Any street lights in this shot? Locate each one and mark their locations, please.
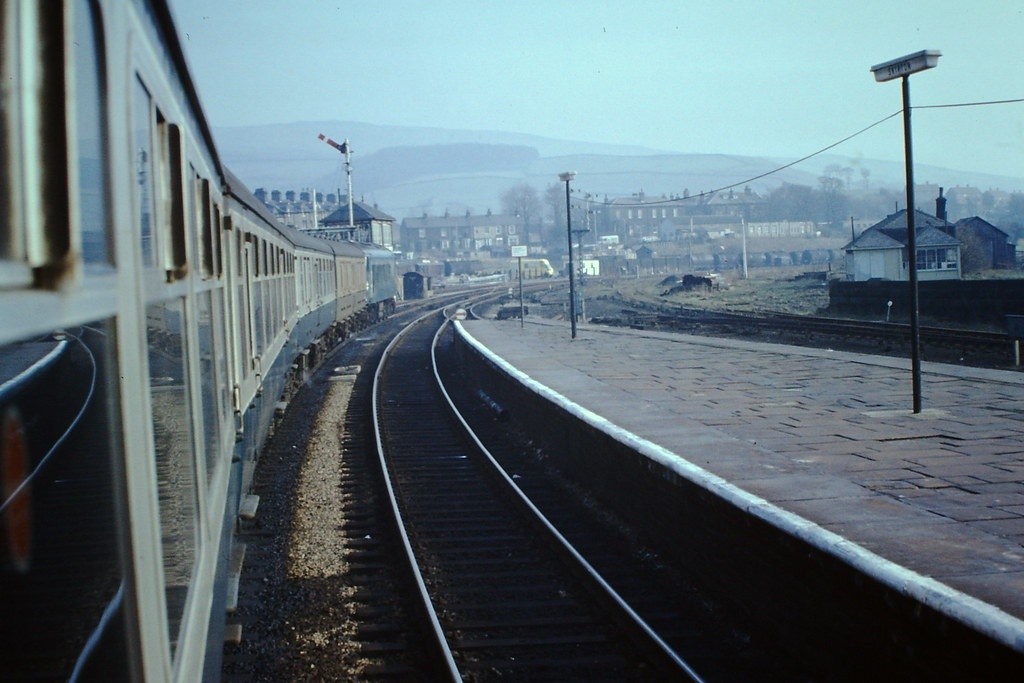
[869,49,944,414]
[557,172,578,340]
